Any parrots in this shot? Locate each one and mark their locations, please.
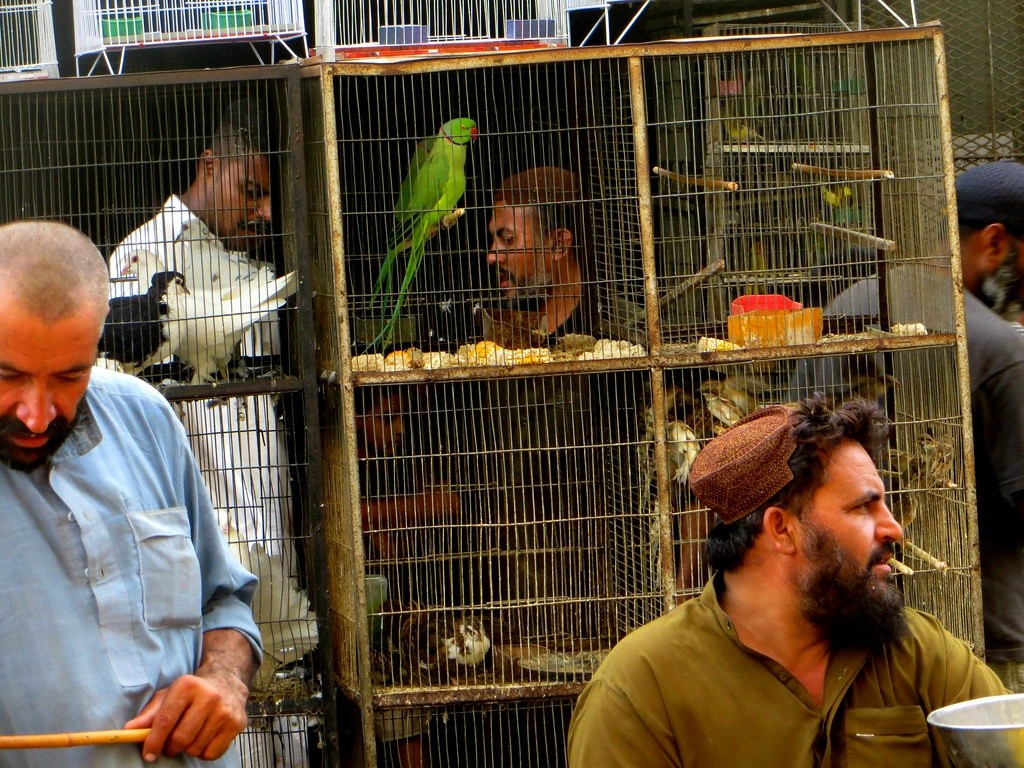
[361,117,476,356]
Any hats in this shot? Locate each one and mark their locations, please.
[495,163,585,209]
[948,158,1024,225]
[687,404,802,527]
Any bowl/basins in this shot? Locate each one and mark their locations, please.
[926,693,1024,768]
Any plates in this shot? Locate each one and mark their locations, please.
[518,649,613,676]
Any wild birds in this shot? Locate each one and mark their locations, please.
[218,514,319,696]
[370,596,527,686]
[818,185,854,209]
[632,375,777,590]
[719,108,766,144]
[827,374,955,541]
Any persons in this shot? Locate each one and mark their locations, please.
[0,220,263,768]
[566,393,1024,768]
[487,167,591,352]
[354,390,462,643]
[93,125,307,767]
[789,162,1024,696]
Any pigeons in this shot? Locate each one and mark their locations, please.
[92,218,296,382]
